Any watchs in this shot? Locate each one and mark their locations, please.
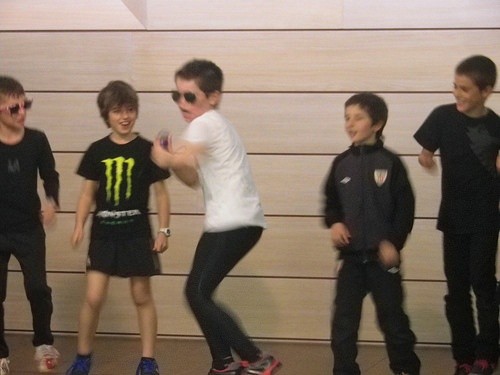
[158,228,171,237]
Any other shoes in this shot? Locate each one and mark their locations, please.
[234,352,283,375]
[136,360,159,375]
[454,360,496,375]
[208,358,239,375]
[0,358,10,375]
[67,357,91,375]
[35,345,59,372]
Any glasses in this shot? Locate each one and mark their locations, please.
[0,98,33,116]
[171,90,200,104]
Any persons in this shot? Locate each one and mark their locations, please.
[0,77,60,375]
[324,94,421,375]
[413,55,500,375]
[152,61,282,375]
[65,80,171,375]
